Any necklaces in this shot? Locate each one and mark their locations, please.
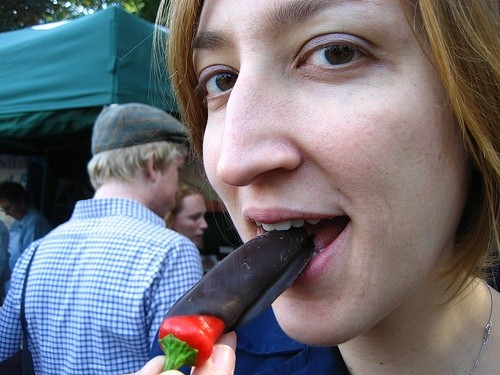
[469,276,494,375]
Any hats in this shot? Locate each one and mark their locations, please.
[91,103,189,155]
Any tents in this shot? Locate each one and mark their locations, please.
[1,6,187,156]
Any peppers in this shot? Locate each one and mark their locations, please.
[159,226,316,373]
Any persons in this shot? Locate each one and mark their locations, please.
[168,183,207,250]
[0,221,10,298]
[0,180,51,281]
[130,0,500,375]
[0,101,199,375]
